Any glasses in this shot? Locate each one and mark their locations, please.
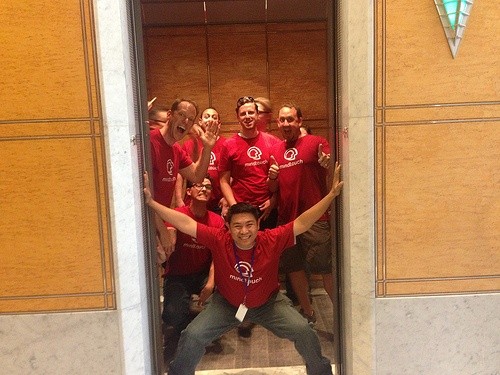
[258,111,271,115]
[189,182,212,190]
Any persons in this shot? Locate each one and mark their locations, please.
[143,160,344,375]
[143,95,344,371]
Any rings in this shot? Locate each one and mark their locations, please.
[325,164,328,167]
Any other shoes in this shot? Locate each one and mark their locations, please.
[299,308,316,323]
[206,339,224,355]
[238,328,252,339]
